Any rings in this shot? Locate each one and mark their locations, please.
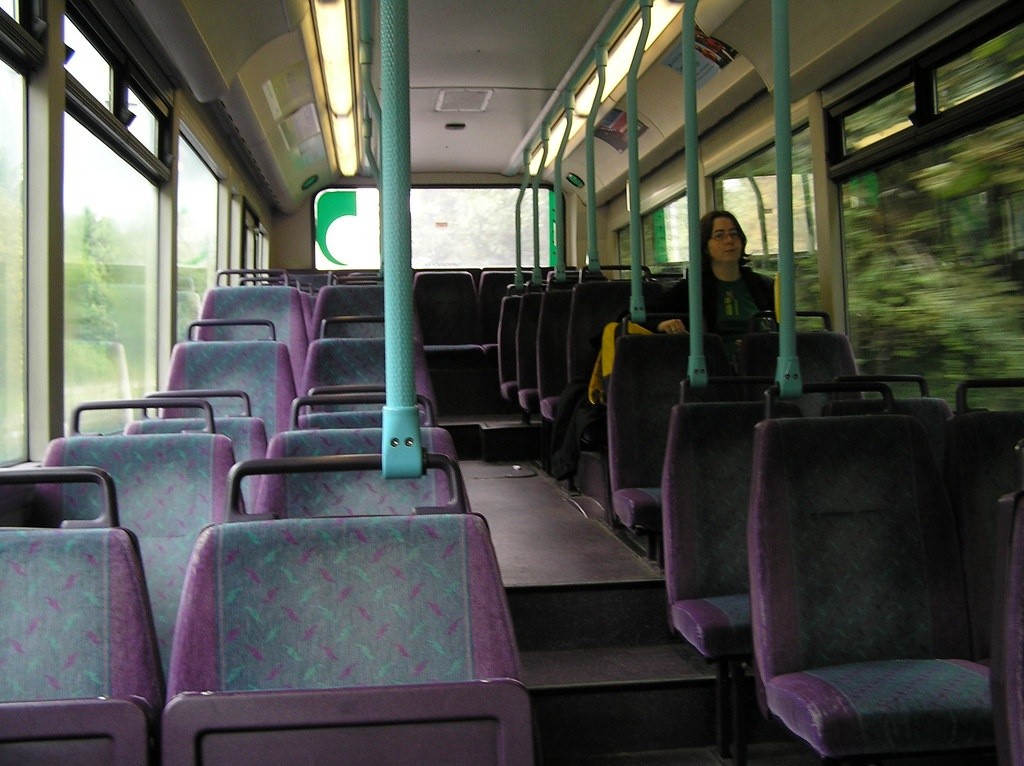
[672,328,677,333]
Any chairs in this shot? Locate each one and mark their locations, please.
[0,268,1023,766]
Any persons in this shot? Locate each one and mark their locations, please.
[649,210,777,336]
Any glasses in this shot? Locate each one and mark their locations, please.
[710,231,740,241]
